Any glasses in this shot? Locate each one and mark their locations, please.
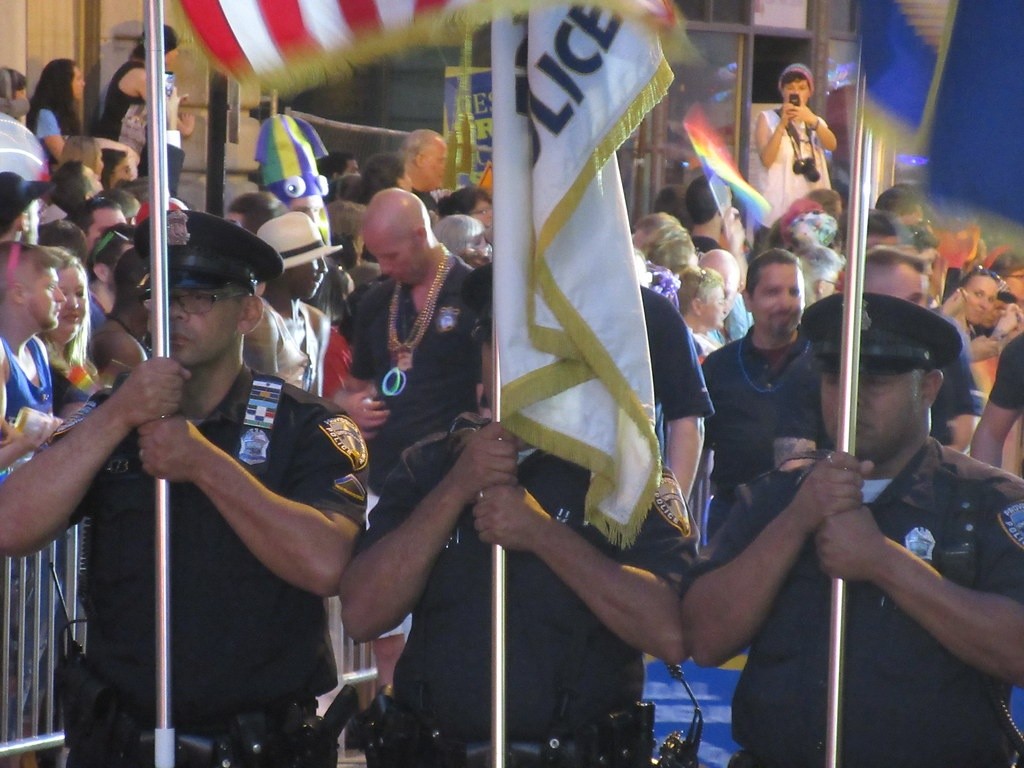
[93,229,130,264]
[970,269,998,280]
[141,294,240,315]
[468,207,493,216]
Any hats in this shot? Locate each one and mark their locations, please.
[254,115,330,204]
[462,264,493,339]
[778,63,815,96]
[0,172,55,226]
[258,211,343,268]
[801,291,963,370]
[134,209,283,296]
[141,25,179,55]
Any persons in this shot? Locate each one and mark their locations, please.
[1,26,1022,767]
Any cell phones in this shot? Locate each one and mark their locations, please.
[165,71,175,98]
[789,94,800,107]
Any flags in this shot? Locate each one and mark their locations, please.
[491,9,675,548]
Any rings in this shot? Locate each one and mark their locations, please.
[140,449,144,457]
[827,454,839,469]
[480,490,484,499]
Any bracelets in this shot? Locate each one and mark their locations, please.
[810,117,821,130]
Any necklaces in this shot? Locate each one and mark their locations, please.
[385,246,455,351]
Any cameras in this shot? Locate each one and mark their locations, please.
[793,158,821,182]
